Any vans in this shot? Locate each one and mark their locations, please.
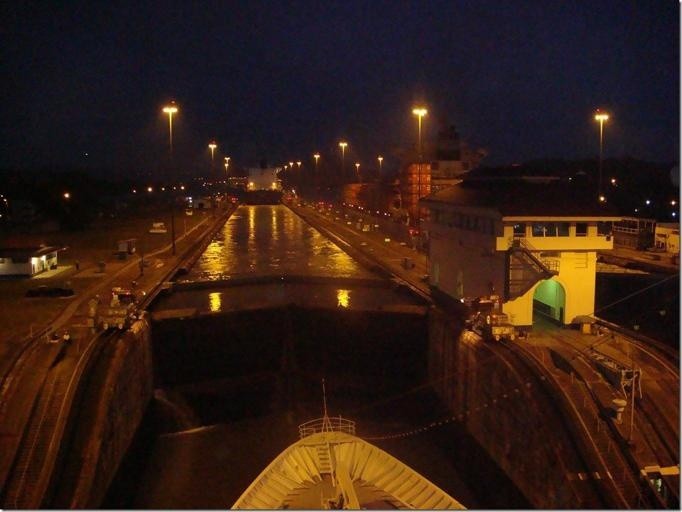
[400,256,417,269]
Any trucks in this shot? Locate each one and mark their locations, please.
[478,312,516,341]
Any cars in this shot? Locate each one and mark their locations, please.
[149,222,168,233]
[297,200,383,233]
[186,209,193,216]
[112,239,137,256]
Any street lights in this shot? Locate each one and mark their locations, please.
[355,161,360,174]
[162,107,177,255]
[224,157,230,172]
[209,138,216,180]
[413,109,427,253]
[314,151,320,166]
[378,155,383,168]
[595,113,610,189]
[276,159,301,176]
[339,143,347,171]
